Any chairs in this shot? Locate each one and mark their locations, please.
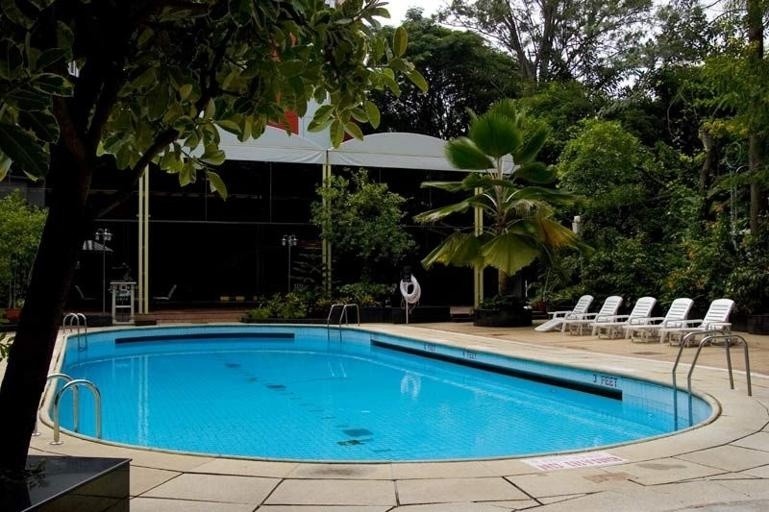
[531,294,735,344]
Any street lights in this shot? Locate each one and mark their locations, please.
[95,228,113,313]
[280,232,298,293]
